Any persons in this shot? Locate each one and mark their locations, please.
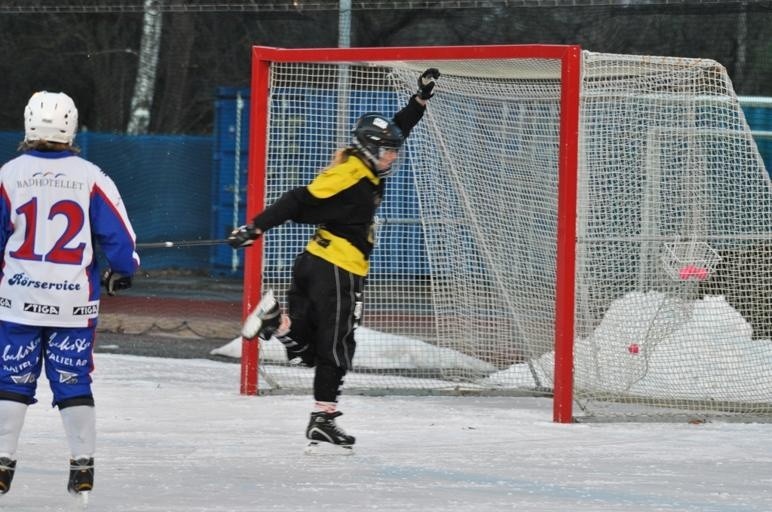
[228,67,440,443]
[0,91,142,493]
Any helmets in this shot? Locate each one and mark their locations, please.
[23,90,78,147]
[350,112,404,178]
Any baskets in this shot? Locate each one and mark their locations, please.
[663,241,721,282]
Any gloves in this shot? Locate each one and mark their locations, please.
[417,69,440,99]
[101,269,131,297]
[228,224,262,248]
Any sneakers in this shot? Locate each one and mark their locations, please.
[252,303,282,340]
[306,411,355,445]
[0,457,17,494]
[68,457,94,494]
